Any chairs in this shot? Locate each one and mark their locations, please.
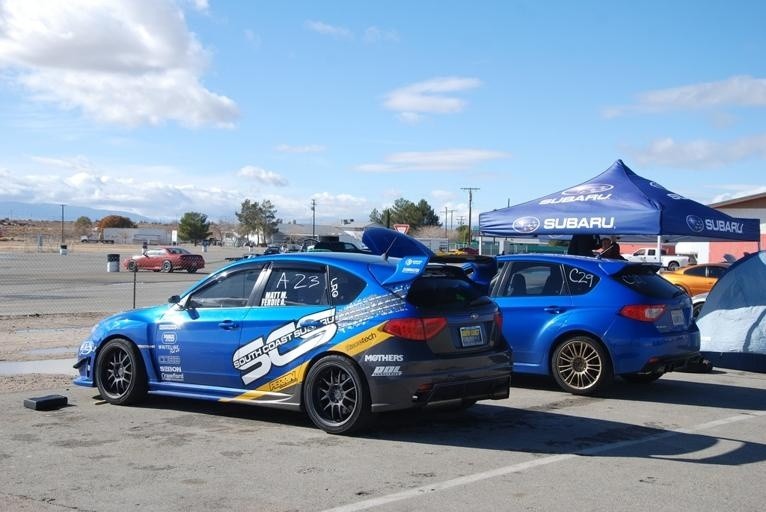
[504,272,557,297]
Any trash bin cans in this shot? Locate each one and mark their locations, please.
[202,244,208,252]
[59,245,67,256]
[106,253,121,272]
[249,246,254,252]
[141,246,147,255]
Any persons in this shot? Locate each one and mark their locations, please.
[596,238,624,260]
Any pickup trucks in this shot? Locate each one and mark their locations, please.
[627,247,697,271]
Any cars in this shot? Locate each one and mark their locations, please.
[462,252,700,395]
[658,263,734,319]
